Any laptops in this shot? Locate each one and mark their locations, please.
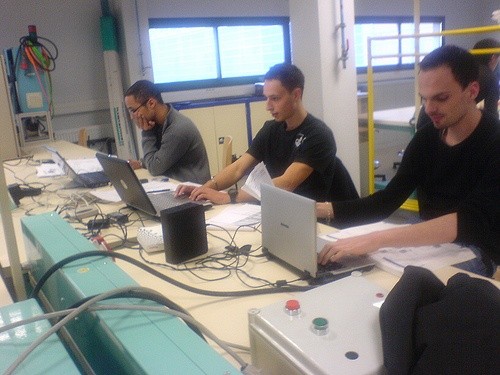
[260,183,375,277]
[41,145,111,187]
[95,152,213,218]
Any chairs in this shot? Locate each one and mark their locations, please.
[78,129,91,146]
[222,137,233,169]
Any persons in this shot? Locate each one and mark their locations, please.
[125,79,211,185]
[315,45,500,279]
[174,64,337,202]
[416,39,500,127]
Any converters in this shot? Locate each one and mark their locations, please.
[88,220,110,230]
[108,211,128,223]
[22,186,41,198]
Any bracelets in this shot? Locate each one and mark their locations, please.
[325,201,331,226]
[212,175,217,191]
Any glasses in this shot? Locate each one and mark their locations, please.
[128,98,150,116]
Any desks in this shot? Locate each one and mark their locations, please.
[0,141,500,368]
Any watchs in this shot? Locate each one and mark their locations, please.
[228,189,238,203]
